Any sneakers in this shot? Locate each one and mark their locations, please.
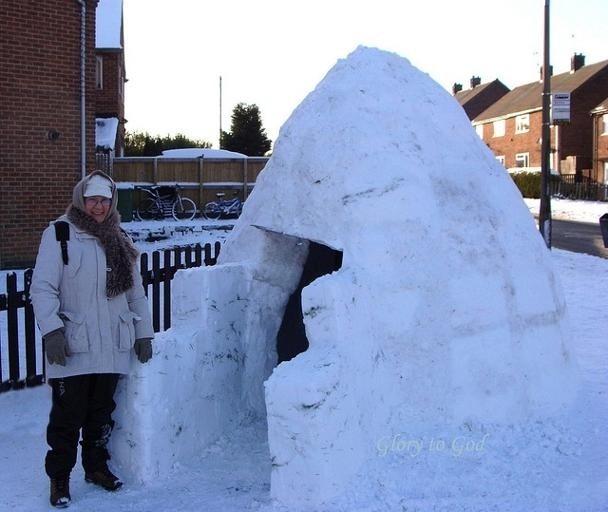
[49,477,71,508]
[84,467,122,491]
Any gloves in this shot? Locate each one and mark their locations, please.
[134,339,151,363]
[43,330,70,366]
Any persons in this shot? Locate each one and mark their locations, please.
[28,169,157,509]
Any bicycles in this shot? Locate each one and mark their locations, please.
[201,188,245,221]
[135,183,197,222]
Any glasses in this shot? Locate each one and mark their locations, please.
[85,198,111,207]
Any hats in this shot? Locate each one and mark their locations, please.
[83,174,113,199]
